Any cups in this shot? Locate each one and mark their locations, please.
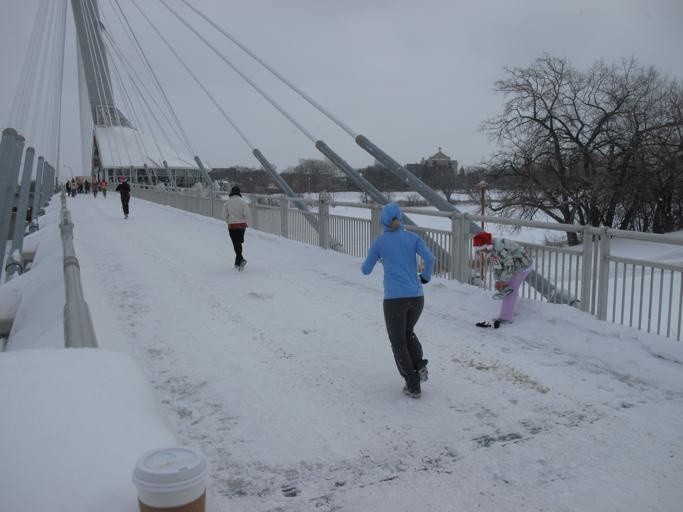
[131,444,209,511]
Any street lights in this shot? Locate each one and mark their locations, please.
[64,165,72,179]
[476,179,491,281]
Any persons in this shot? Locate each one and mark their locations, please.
[115,176,130,219]
[65,176,107,198]
[471,231,533,325]
[221,186,247,271]
[361,202,434,398]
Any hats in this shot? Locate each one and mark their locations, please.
[228,186,242,198]
[472,232,493,251]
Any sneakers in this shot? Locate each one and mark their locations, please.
[491,286,513,300]
[238,259,247,271]
[418,368,429,382]
[491,318,513,324]
[403,386,421,398]
